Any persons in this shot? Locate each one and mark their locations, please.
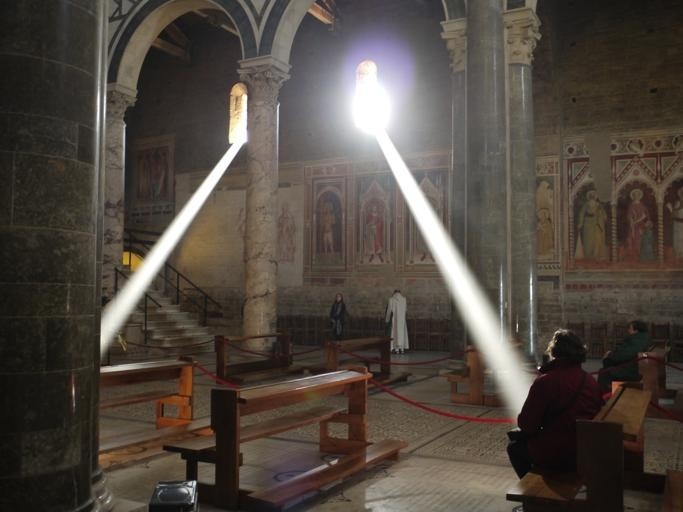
[329,293,345,340]
[385,290,410,354]
[277,202,297,262]
[320,205,336,253]
[536,180,682,260]
[506,328,606,478]
[367,205,382,252]
[598,320,651,399]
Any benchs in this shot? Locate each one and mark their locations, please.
[162,363,409,512]
[448,345,506,407]
[214,328,304,386]
[507,385,683,512]
[611,346,683,420]
[99,355,214,469]
[303,336,409,397]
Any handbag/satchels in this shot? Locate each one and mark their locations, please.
[508,428,529,441]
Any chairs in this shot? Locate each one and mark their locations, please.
[567,320,683,362]
[277,315,450,351]
